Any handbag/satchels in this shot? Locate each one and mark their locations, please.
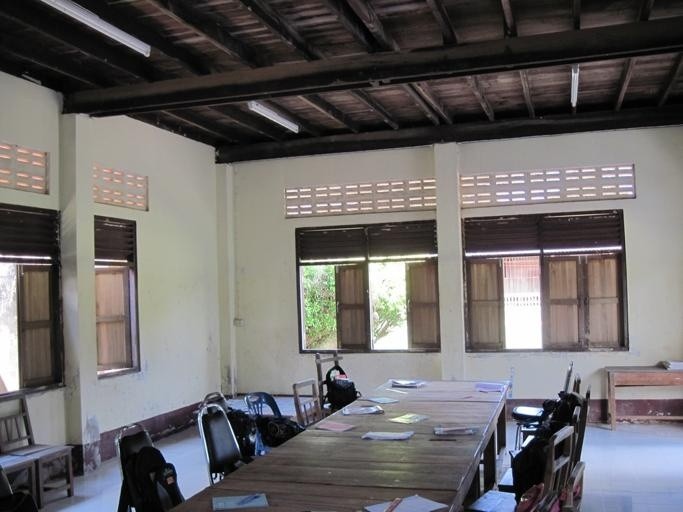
[132,445,182,512]
[508,391,583,512]
[256,413,304,447]
[327,365,357,409]
[227,409,262,458]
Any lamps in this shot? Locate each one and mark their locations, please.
[42,0,151,58]
[571,64,579,107]
[248,101,299,134]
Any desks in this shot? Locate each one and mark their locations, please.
[167,380,513,512]
[604,366,683,430]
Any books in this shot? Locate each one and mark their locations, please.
[211,491,270,511]
[360,430,415,441]
[342,405,384,415]
[432,424,478,441]
[473,381,504,392]
[662,359,682,372]
[388,413,430,424]
[314,420,353,433]
[364,495,450,512]
[390,378,427,388]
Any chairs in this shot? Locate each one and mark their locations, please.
[0,454,38,512]
[509,360,574,450]
[496,378,590,491]
[468,423,576,509]
[114,423,187,511]
[197,404,246,491]
[245,391,283,456]
[519,376,582,441]
[202,390,263,467]
[314,351,359,417]
[0,392,75,508]
[292,380,331,431]
